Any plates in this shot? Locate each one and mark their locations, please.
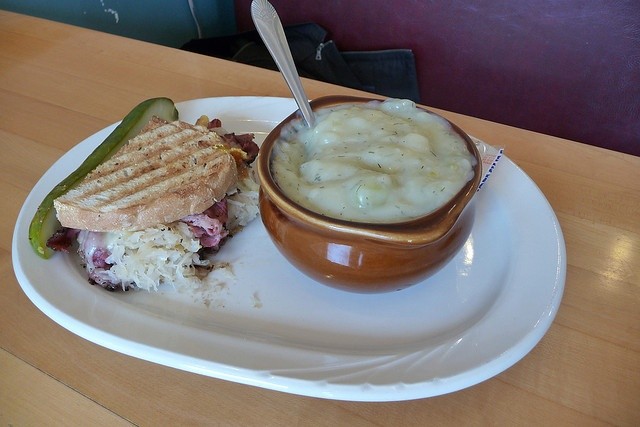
[10,94,569,404]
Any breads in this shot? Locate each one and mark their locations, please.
[53,119,235,231]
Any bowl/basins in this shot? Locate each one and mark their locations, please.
[256,97,481,296]
